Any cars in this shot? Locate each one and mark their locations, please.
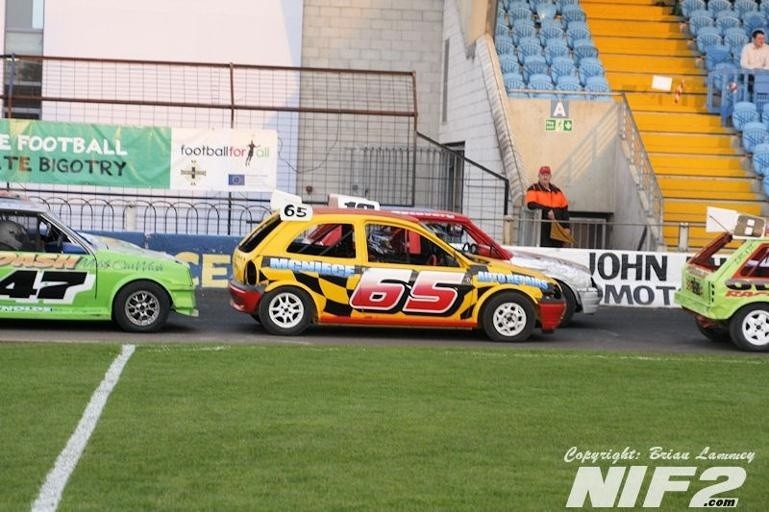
[228,189,564,343]
[677,234,769,351]
[0,190,201,334]
[325,194,603,325]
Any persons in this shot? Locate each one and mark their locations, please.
[1,219,46,254]
[367,230,399,263]
[526,165,572,247]
[737,30,768,96]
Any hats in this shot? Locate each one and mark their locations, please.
[539,166,551,175]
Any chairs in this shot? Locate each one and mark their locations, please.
[495,0,614,101]
[681,0,769,201]
